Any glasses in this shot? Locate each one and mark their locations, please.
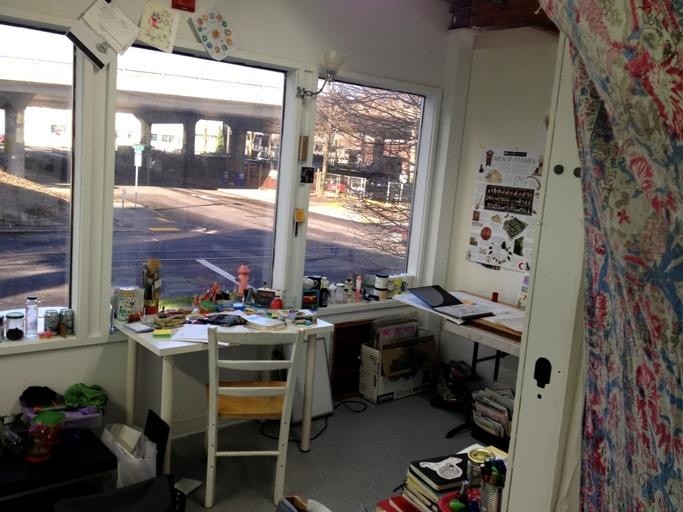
[295,50,346,102]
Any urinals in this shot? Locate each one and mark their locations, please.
[153,329,172,338]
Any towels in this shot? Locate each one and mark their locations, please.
[221,171,245,186]
[104,423,153,486]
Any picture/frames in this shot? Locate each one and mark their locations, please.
[113,308,336,476]
[442,295,523,439]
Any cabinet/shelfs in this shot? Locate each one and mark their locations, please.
[335,278,357,304]
[25,295,39,340]
[466,449,496,487]
[6,311,24,341]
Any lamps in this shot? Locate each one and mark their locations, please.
[203,322,307,508]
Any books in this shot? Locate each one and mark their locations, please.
[354,334,435,406]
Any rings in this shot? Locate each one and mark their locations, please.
[480,458,505,493]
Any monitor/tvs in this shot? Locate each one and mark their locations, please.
[472,383,514,438]
[171,314,287,346]
[111,424,148,458]
[408,285,496,320]
[376,443,469,512]
